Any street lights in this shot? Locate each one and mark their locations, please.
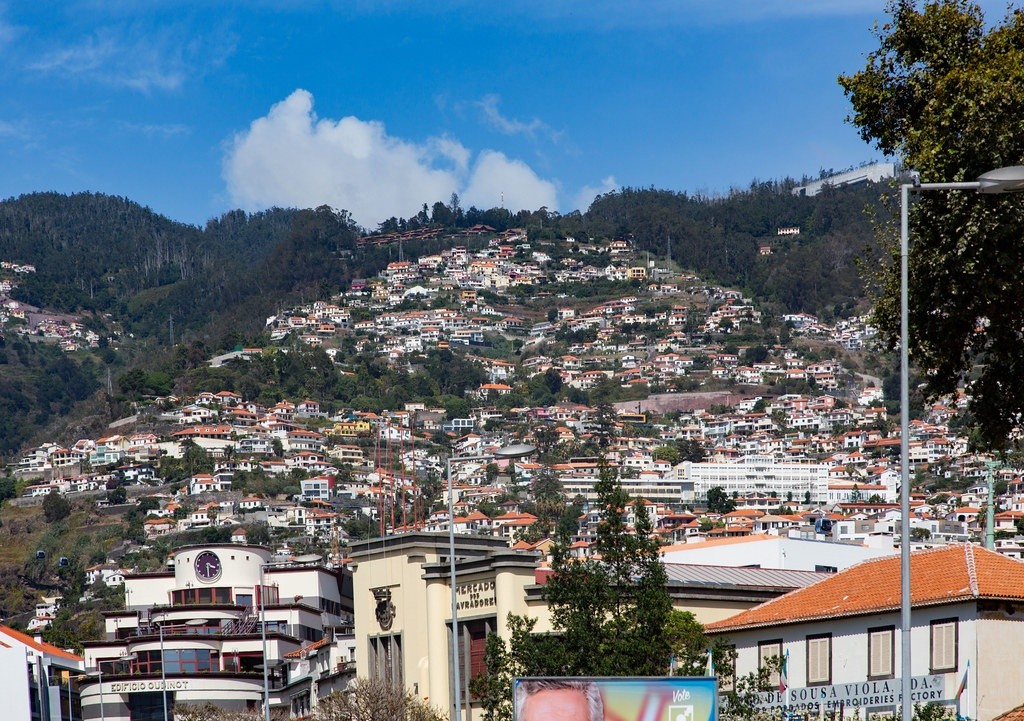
[901,164,1024,721]
[99,656,137,721]
[160,619,209,721]
[259,555,324,721]
[68,671,104,721]
[448,445,538,721]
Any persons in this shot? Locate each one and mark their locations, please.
[517,679,603,721]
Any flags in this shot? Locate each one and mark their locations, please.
[954,670,967,700]
[779,658,787,694]
